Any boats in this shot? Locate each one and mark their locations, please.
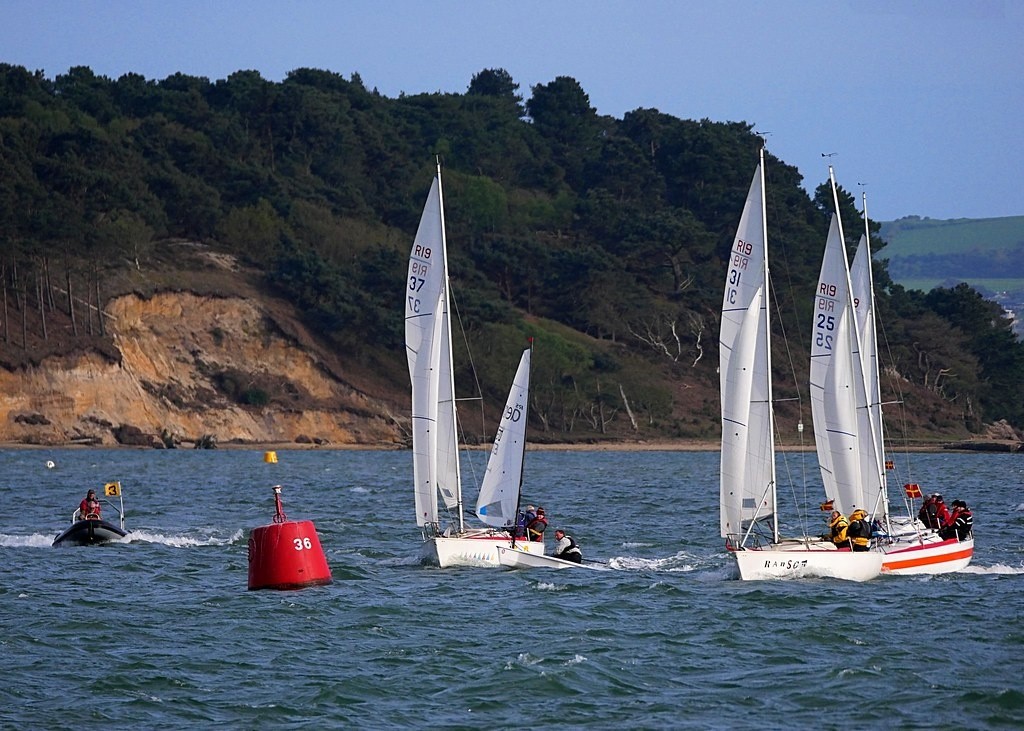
[50,499,135,549]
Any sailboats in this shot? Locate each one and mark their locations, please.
[474,334,615,574]
[405,152,548,567]
[717,140,887,582]
[808,157,976,579]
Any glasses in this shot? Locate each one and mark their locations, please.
[936,499,942,502]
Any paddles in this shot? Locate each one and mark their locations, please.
[544,553,607,564]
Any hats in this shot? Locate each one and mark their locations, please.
[958,501,966,509]
[951,500,960,506]
[537,507,544,515]
[932,492,940,498]
[526,505,535,511]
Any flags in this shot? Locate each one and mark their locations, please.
[904,484,923,498]
[105,482,121,496]
[820,500,833,511]
[885,461,895,470]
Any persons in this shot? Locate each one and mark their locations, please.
[518,509,527,528]
[79,489,101,519]
[939,499,973,541]
[919,492,950,528]
[525,507,547,541]
[525,505,537,526]
[823,511,850,548]
[552,529,582,564]
[846,510,872,552]
